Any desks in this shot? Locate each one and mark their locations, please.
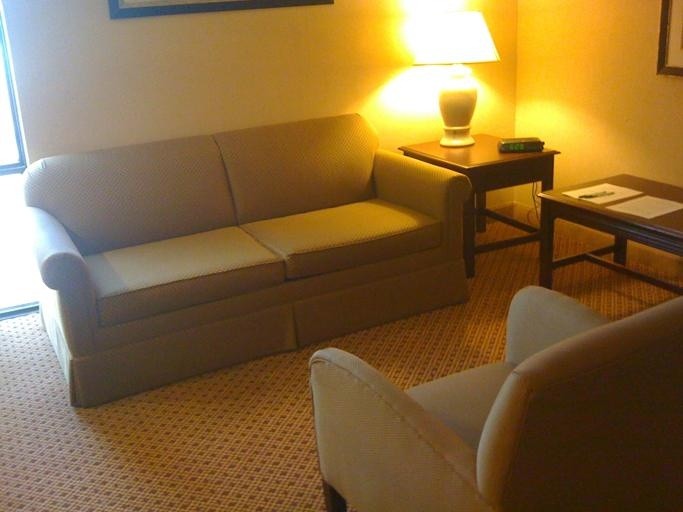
[399,132,560,279]
[537,175,683,296]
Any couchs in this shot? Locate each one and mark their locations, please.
[309,286,682,512]
[22,113,472,409]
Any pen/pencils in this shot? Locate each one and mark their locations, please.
[589,192,615,197]
[579,190,607,198]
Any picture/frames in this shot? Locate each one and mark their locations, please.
[107,0,333,19]
[655,0,683,76]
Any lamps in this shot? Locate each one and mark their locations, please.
[413,12,501,148]
